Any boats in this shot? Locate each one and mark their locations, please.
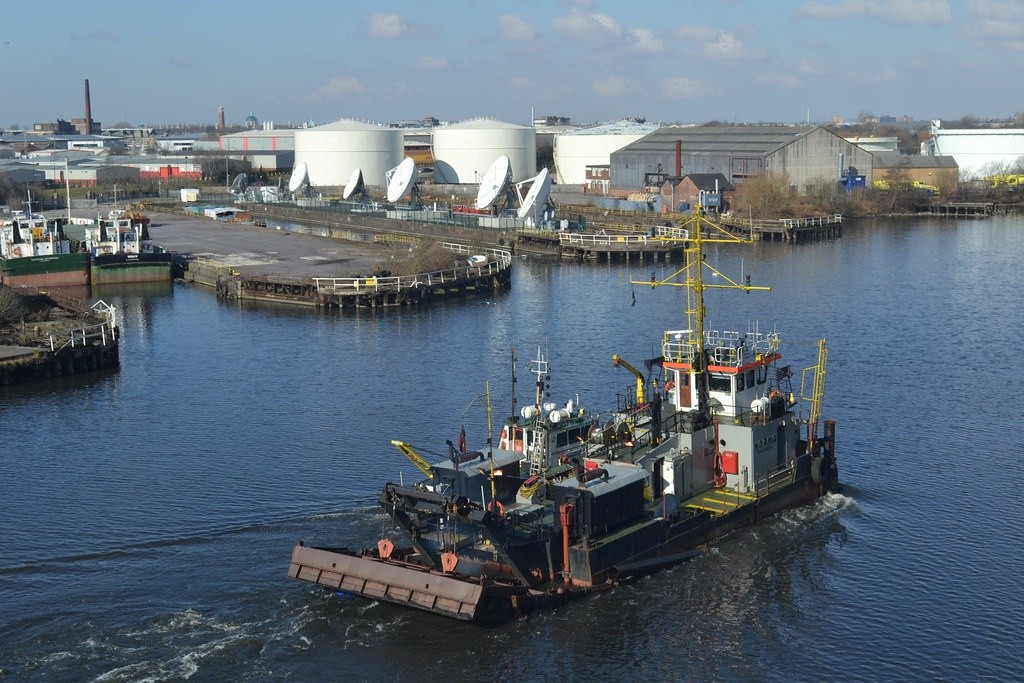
[0,189,90,290]
[48,155,96,226]
[285,189,843,625]
[83,182,175,284]
